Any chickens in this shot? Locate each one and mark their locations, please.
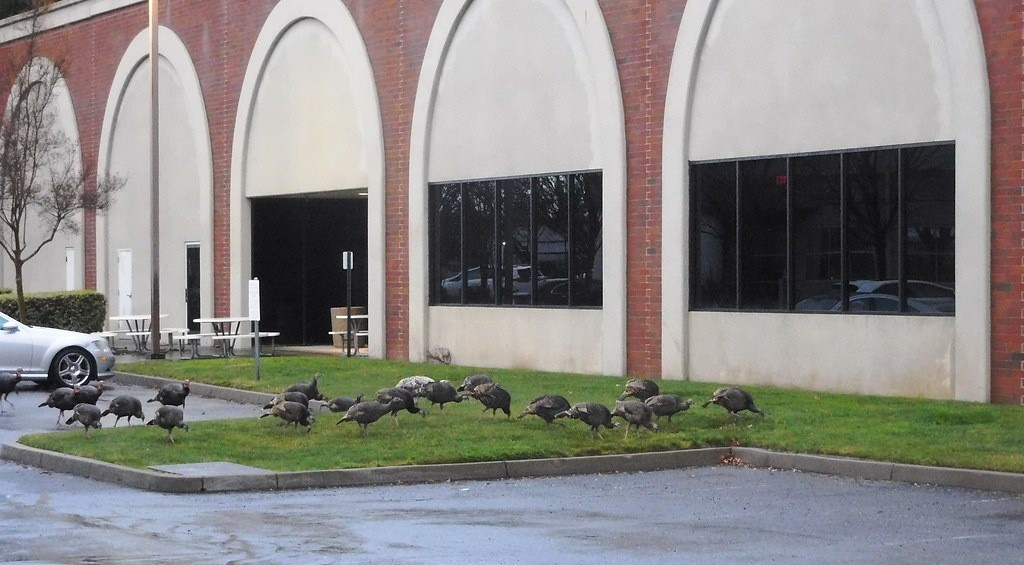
[38,379,191,444]
[702,386,765,418]
[0,367,24,411]
[257,371,697,440]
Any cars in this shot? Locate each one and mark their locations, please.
[0,312,116,390]
[502,279,603,306]
[793,293,944,315]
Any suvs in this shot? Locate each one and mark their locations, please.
[440,266,545,299]
[833,278,955,308]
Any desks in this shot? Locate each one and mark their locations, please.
[336,315,368,356]
[109,314,170,353]
[193,317,249,357]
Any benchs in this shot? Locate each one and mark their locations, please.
[92,328,280,339]
[328,330,369,336]
